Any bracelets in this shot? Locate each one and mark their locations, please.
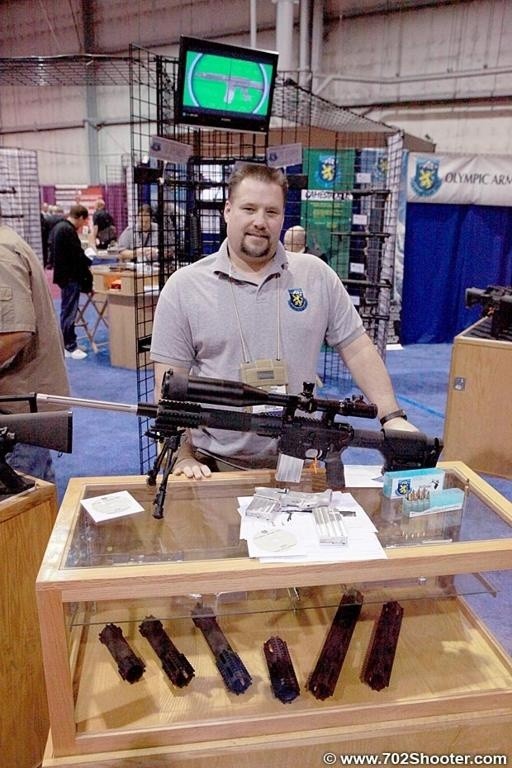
[376,405,412,425]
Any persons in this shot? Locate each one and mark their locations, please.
[40,203,160,264]
[50,204,99,355]
[0,221,72,483]
[151,161,423,480]
[282,225,329,265]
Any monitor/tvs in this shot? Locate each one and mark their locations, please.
[173,35,279,136]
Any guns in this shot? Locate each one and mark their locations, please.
[30,370,442,516]
[0,394,73,458]
[185,68,270,104]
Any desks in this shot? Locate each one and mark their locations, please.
[442,314,511,480]
[108,290,158,371]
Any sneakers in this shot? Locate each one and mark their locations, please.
[77,345,87,351]
[64,348,87,359]
